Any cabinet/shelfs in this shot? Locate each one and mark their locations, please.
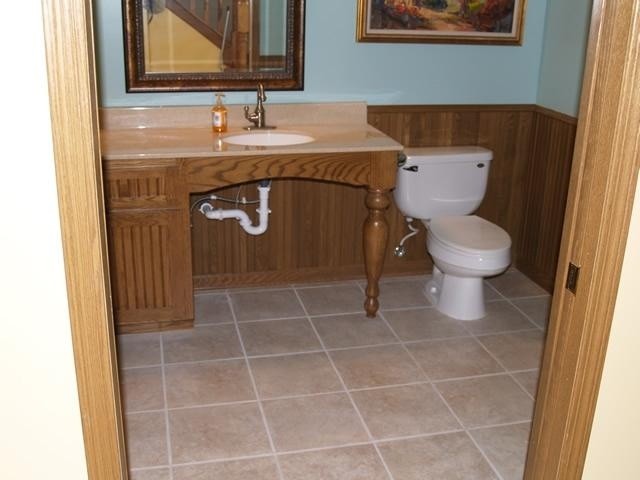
[103,158,194,335]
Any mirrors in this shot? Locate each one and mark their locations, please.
[120,1,306,93]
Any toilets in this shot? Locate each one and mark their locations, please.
[393,146,513,321]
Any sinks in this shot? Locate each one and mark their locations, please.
[218,129,317,147]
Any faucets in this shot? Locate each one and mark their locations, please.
[245,83,267,128]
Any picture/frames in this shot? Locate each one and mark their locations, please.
[356,0,526,46]
